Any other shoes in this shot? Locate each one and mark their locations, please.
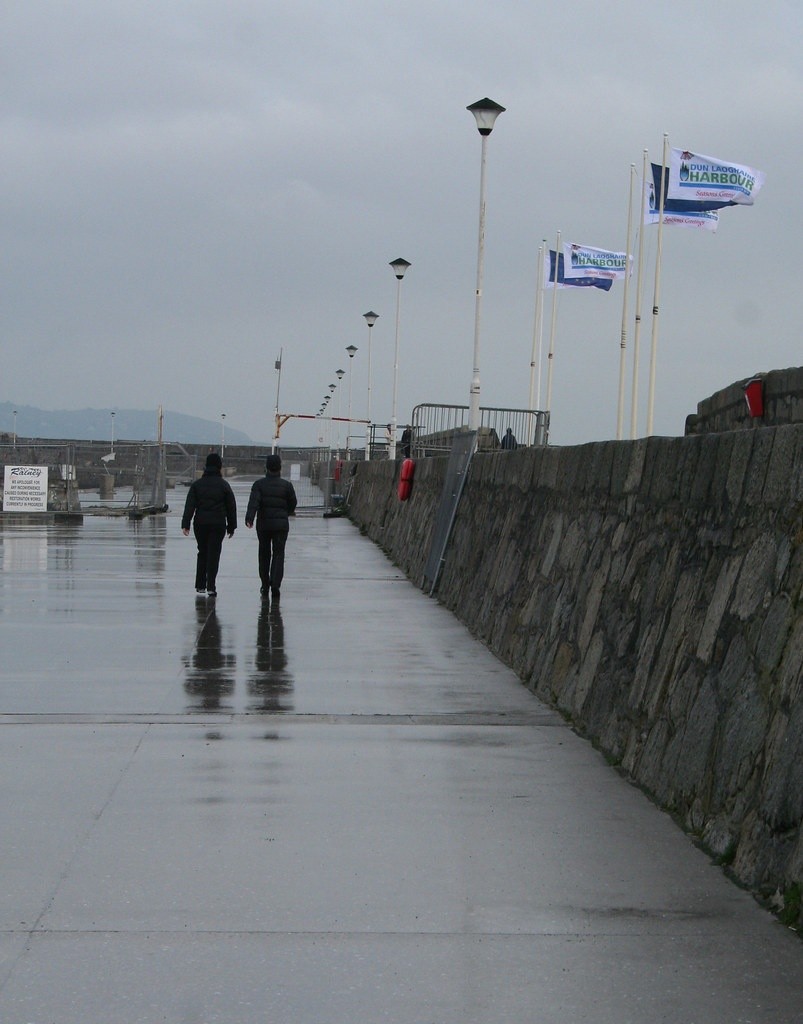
[196,588,206,593]
[260,587,269,596]
[207,587,217,595]
[271,583,280,597]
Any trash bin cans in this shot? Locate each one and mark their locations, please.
[331,494,344,512]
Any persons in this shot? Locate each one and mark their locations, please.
[402,424,413,458]
[489,429,499,449]
[501,428,518,449]
[385,424,398,454]
[245,455,297,597]
[181,454,236,596]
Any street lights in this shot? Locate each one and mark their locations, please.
[220,413,226,457]
[363,309,381,461]
[110,411,115,445]
[314,342,359,461]
[12,410,18,446]
[387,257,413,459]
[465,96,508,431]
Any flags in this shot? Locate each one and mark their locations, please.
[562,241,634,280]
[649,162,669,212]
[544,249,613,292]
[642,181,720,232]
[663,146,765,211]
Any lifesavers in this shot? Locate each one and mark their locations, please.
[298,449,304,455]
[335,459,343,481]
[319,437,324,442]
[398,459,413,500]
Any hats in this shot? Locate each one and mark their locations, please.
[205,453,222,469]
[266,455,281,472]
